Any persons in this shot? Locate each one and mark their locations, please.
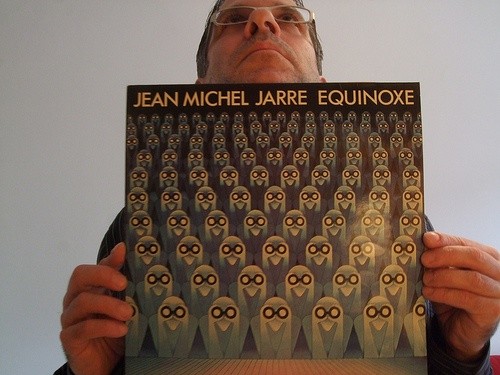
[51,0,500,375]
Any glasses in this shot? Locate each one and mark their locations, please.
[205,4,317,58]
